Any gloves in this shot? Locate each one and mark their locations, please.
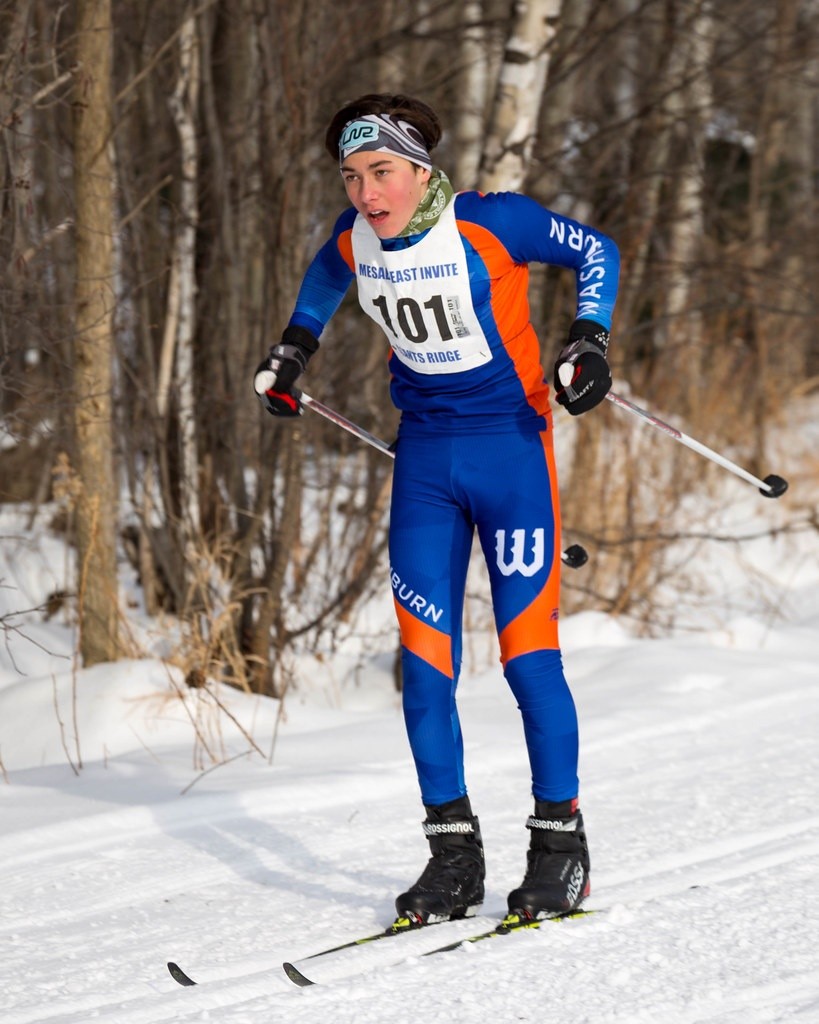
[554,319,613,416]
[253,326,319,417]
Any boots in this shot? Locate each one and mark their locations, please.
[395,795,486,924]
[507,796,590,918]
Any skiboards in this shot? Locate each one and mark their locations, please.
[167,908,605,988]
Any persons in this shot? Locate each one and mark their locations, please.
[255,95,620,917]
